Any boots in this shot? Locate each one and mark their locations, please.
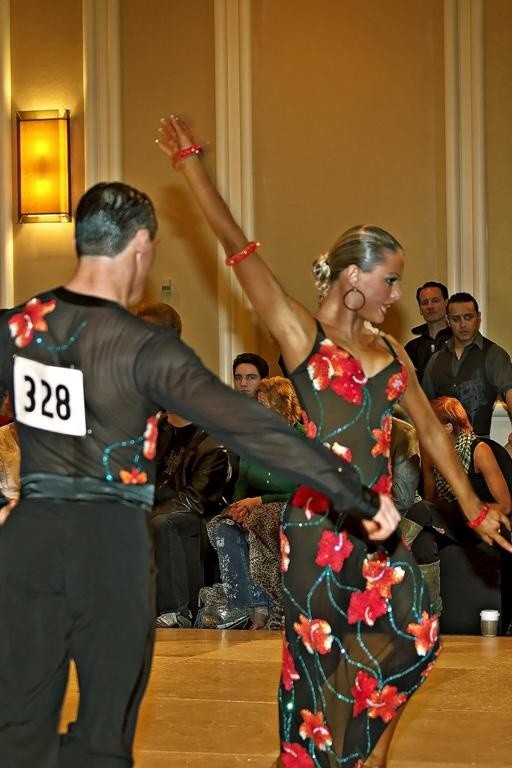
[398,516,424,548]
[417,558,443,616]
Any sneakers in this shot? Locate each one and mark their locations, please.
[155,608,192,628]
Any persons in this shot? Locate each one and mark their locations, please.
[1,182,404,768]
[154,113,512,767]
[1,281,511,639]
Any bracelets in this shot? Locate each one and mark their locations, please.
[170,140,208,171]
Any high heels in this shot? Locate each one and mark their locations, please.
[216,614,252,629]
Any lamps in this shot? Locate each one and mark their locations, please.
[16,108,73,224]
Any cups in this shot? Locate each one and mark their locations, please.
[480,610,500,637]
